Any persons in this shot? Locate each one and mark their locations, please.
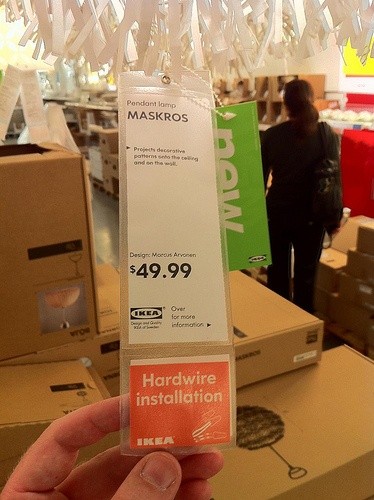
[257,80,341,314]
[0,395,224,500]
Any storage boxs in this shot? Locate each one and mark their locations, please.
[0,143,100,362]
[241,215,374,345]
[225,270,325,397]
[0,262,121,399]
[206,345,374,500]
[89,128,119,194]
[0,357,113,490]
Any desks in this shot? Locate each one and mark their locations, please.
[6,89,114,135]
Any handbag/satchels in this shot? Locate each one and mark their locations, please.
[309,121,342,232]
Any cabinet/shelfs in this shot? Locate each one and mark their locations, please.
[212,76,327,133]
